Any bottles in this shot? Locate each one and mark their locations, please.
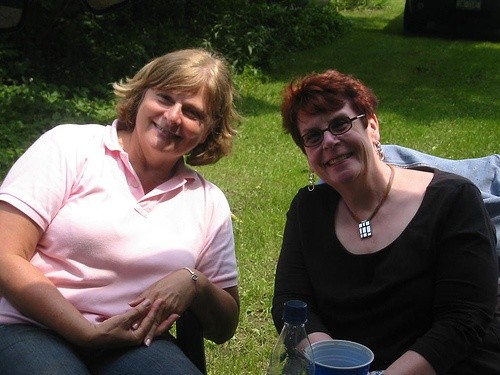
[267,300,315,375]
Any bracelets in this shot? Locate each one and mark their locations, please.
[185,267,200,292]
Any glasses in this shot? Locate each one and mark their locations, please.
[299,113,367,147]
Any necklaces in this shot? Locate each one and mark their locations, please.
[344,163,396,240]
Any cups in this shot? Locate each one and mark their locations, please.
[306,340,374,375]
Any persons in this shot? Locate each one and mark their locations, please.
[272,71,500,375]
[0,47,244,375]
